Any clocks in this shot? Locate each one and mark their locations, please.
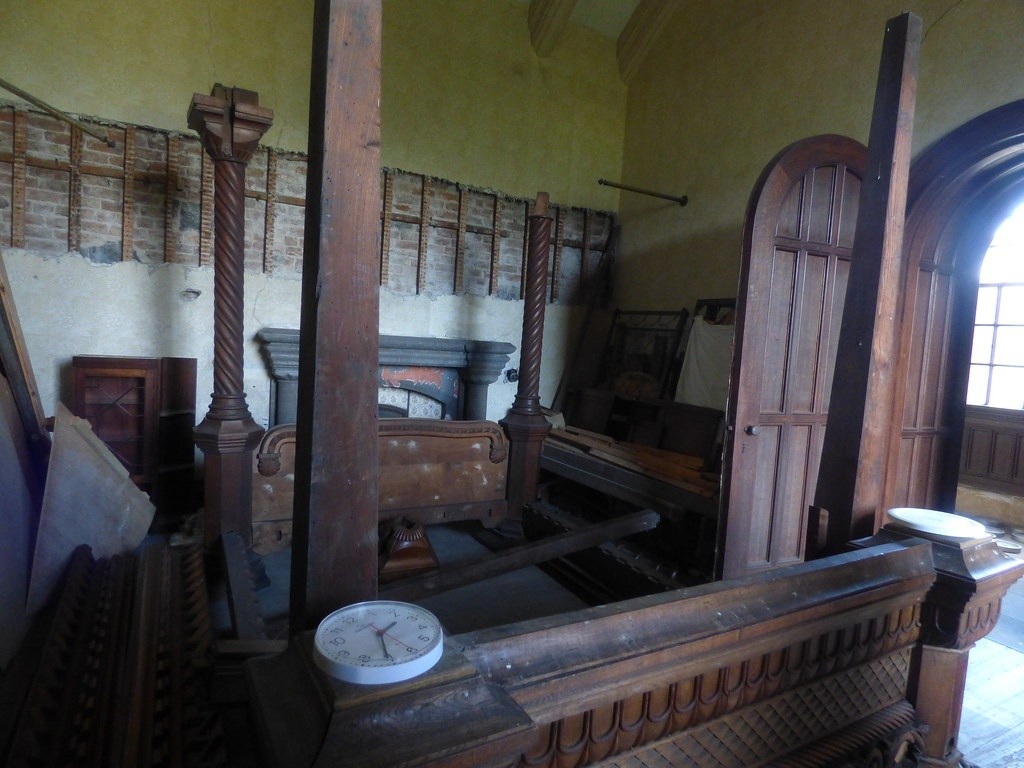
[314,599,444,686]
